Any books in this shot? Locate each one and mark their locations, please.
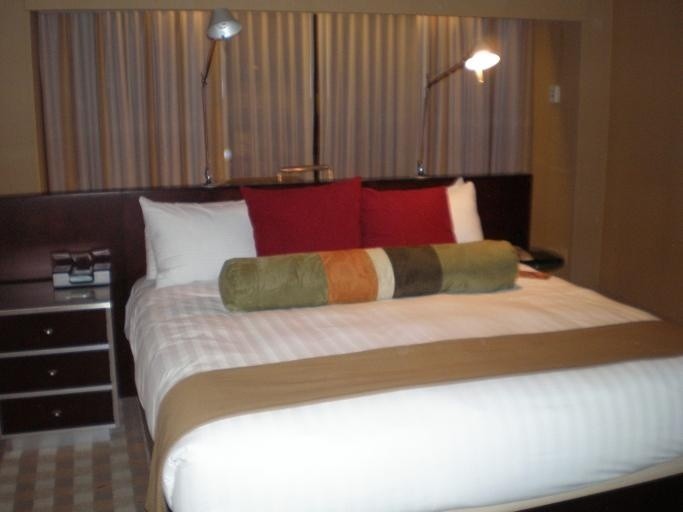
[513,245,536,262]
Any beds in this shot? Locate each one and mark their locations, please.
[1,174,683,512]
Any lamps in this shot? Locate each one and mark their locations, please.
[415,39,501,176]
[198,7,243,189]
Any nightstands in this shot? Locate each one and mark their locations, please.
[2,279,122,450]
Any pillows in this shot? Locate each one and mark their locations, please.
[138,177,519,312]
